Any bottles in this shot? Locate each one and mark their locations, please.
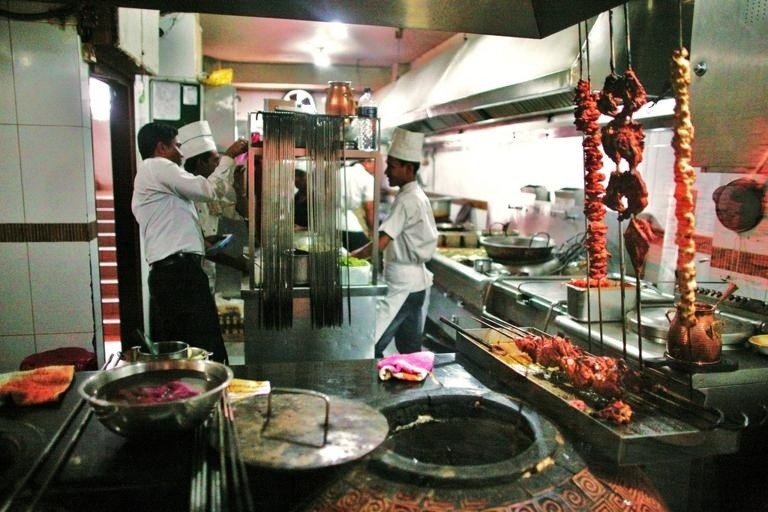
[357,87,377,151]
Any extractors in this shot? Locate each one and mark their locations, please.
[371,0,687,142]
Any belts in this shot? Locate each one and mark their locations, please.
[166,253,202,261]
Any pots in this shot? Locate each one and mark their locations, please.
[713,176,768,234]
[189,347,213,361]
[284,248,310,288]
[430,197,452,222]
[479,222,557,262]
[137,340,190,360]
[437,222,465,231]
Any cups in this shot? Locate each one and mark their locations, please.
[474,259,491,273]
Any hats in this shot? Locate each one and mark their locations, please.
[387,128,425,162]
[178,120,216,160]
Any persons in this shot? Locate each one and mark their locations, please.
[347,126,438,360]
[236,148,384,273]
[131,121,249,363]
[177,119,248,295]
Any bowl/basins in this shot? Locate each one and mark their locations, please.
[77,357,234,439]
[748,334,768,355]
